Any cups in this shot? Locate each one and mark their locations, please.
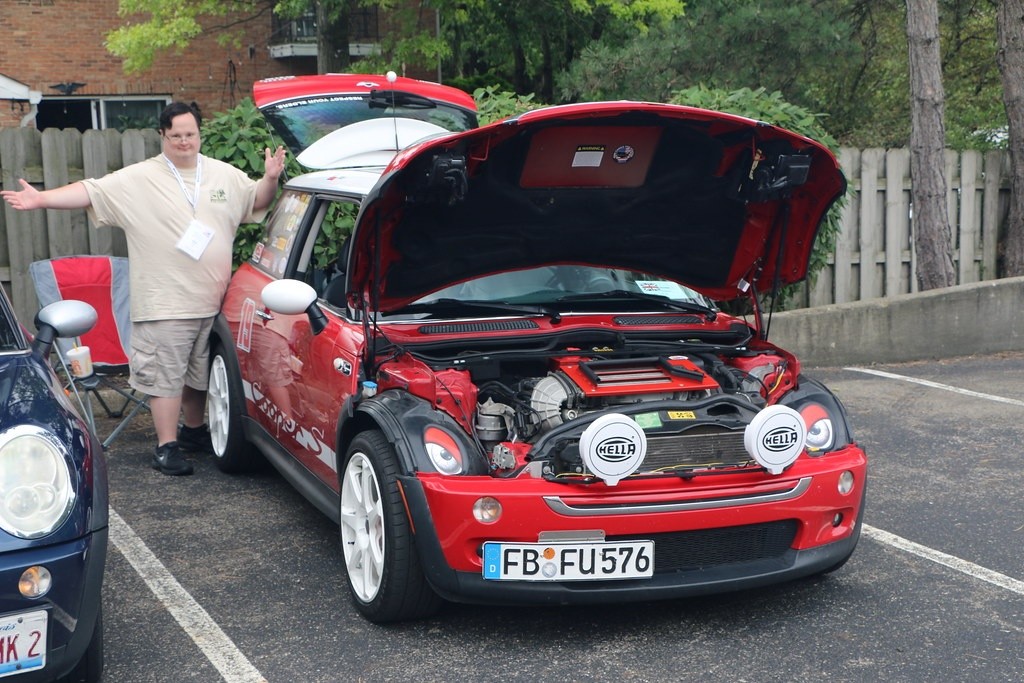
[66,346,94,379]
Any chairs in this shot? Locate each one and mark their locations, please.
[30,255,183,452]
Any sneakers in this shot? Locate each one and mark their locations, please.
[152,441,194,475]
[177,423,214,452]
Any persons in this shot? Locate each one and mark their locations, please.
[0,101,286,475]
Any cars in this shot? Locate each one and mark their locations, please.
[205,70,869,622]
[0,282,109,682]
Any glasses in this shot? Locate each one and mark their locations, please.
[162,132,201,143]
